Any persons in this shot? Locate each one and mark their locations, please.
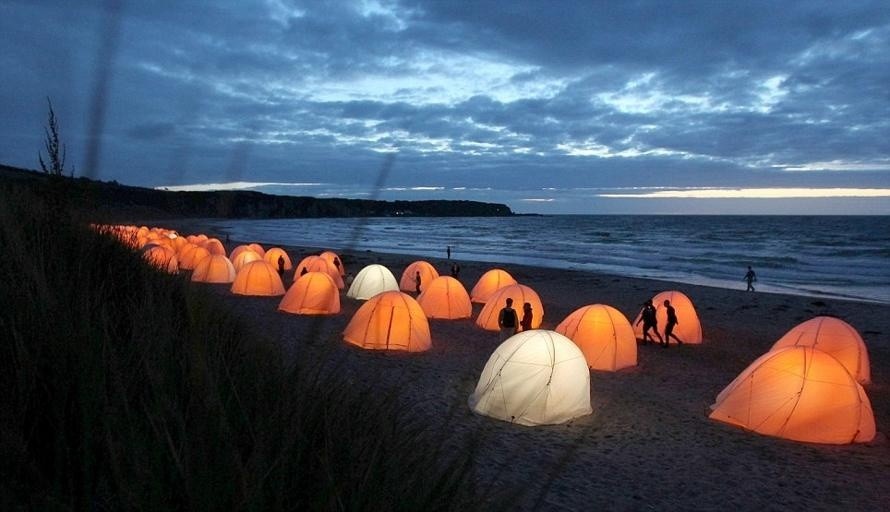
[520,302,533,333]
[645,299,663,344]
[636,303,656,344]
[446,245,451,258]
[741,266,756,292]
[333,257,340,269]
[450,259,460,277]
[498,298,519,344]
[416,271,422,294]
[664,300,684,345]
[301,267,307,276]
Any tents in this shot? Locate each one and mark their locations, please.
[319,251,346,276]
[708,344,875,448]
[346,264,399,301]
[769,315,873,387]
[277,272,342,317]
[340,291,433,354]
[474,284,545,332]
[88,222,294,297]
[633,290,704,347]
[415,276,473,320]
[293,255,345,292]
[470,269,519,304]
[553,304,638,371]
[467,329,594,427]
[400,260,440,294]
